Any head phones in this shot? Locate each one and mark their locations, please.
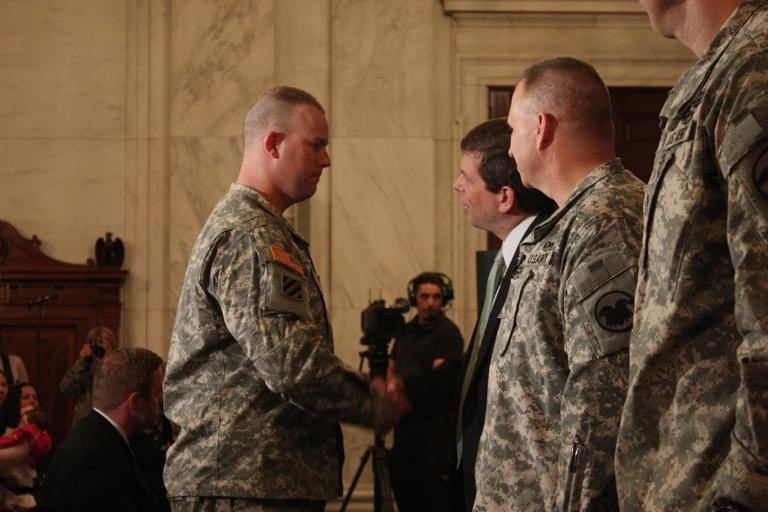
[408,273,454,307]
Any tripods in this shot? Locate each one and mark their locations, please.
[341,342,405,512]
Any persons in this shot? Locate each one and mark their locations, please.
[456,122,558,511]
[160,88,400,512]
[385,272,469,512]
[470,56,650,511]
[614,1,768,512]
[0,326,174,511]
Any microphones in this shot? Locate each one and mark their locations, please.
[430,302,452,317]
[28,292,59,304]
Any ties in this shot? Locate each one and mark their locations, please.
[455,250,504,472]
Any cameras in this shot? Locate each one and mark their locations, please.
[86,345,105,363]
[28,409,49,429]
